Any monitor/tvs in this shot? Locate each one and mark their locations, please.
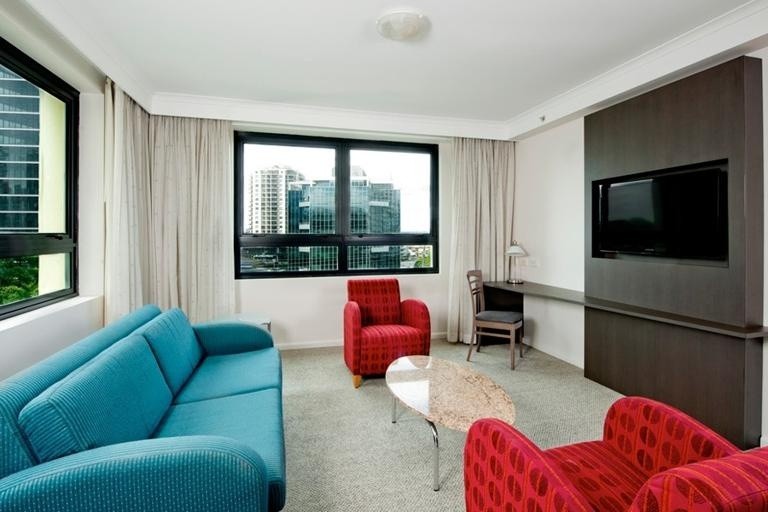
[597,168,727,260]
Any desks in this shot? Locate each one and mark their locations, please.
[477,279,768,451]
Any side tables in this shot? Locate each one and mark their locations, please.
[213,313,271,333]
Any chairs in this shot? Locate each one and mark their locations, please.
[466,270,524,370]
[344,278,430,388]
[463,395,768,512]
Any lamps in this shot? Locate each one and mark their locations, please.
[504,241,525,284]
[377,11,431,42]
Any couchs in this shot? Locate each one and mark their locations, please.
[0,305,285,512]
